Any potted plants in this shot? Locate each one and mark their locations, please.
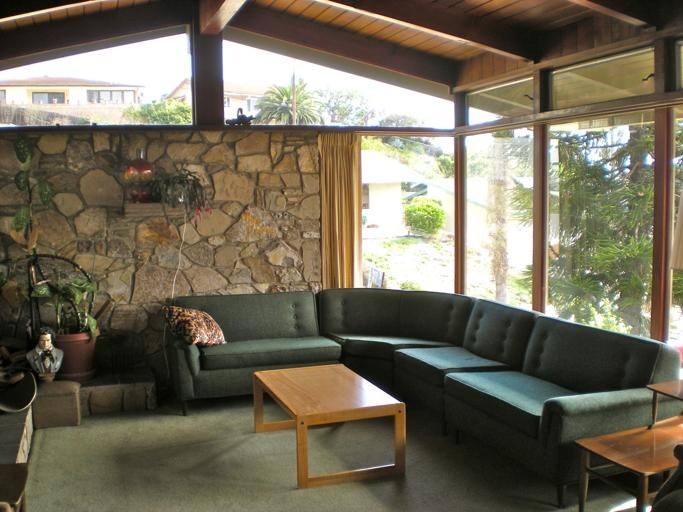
[150,167,210,227]
[34,271,111,374]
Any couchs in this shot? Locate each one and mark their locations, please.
[159,288,347,415]
[390,295,683,511]
[313,282,479,419]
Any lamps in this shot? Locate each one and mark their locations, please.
[123,154,154,204]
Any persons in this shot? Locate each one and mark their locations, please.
[25,325,64,374]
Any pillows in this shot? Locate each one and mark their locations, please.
[161,298,228,350]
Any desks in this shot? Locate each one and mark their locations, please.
[250,364,411,492]
[571,370,683,511]
